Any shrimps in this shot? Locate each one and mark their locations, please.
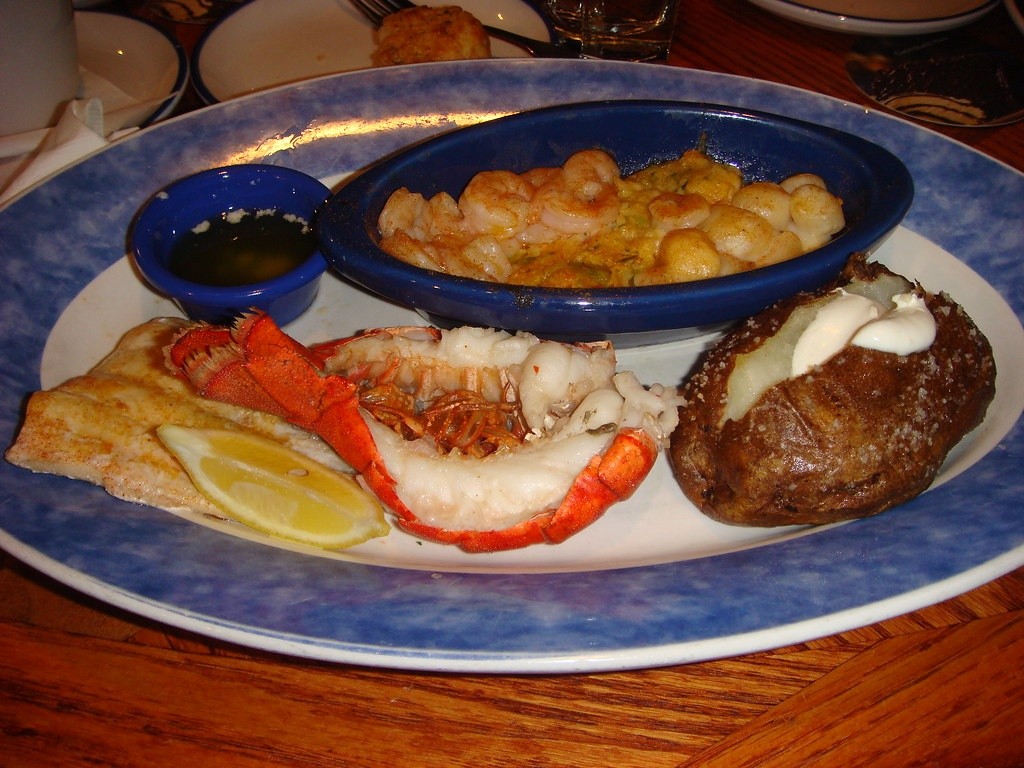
[375,148,847,288]
[161,303,684,554]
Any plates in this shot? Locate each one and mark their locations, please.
[321,101,913,351]
[1004,0,1024,29]
[192,0,559,107]
[0,58,1024,677]
[844,38,1023,127]
[150,0,226,24]
[0,7,190,128]
[754,0,1001,35]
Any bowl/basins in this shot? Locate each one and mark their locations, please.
[128,164,332,327]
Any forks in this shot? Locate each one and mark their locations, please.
[348,0,559,59]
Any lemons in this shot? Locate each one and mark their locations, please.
[157,424,393,549]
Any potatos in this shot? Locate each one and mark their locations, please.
[667,262,998,526]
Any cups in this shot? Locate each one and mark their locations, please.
[0,0,82,138]
[541,0,680,65]
